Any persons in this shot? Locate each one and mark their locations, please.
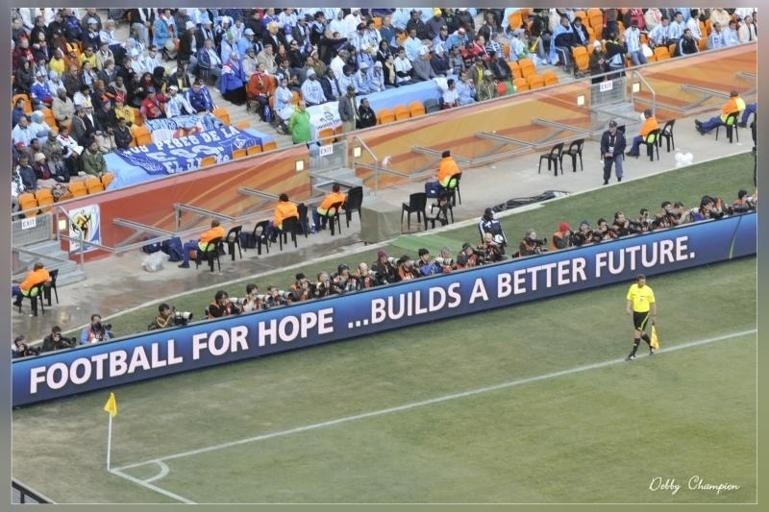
[11,6,758,358]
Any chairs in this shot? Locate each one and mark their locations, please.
[42,268,60,304]
[18,281,46,316]
